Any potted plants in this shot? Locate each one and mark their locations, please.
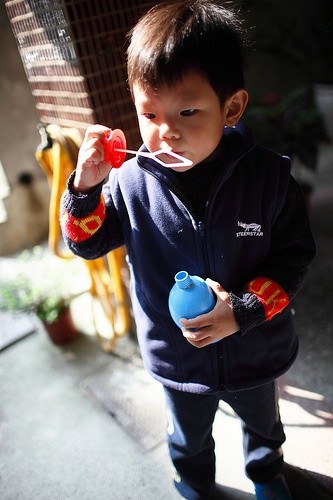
[1,274,79,346]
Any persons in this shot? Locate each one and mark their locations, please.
[59,0,318,500]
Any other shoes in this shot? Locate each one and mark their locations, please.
[254,473,292,500]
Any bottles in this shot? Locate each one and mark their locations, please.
[168,272,221,343]
